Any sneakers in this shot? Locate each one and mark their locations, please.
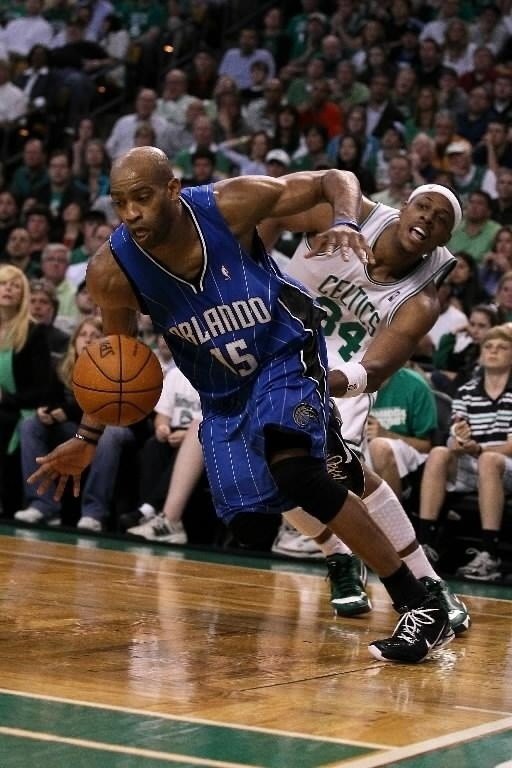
[322,550,371,618]
[364,594,451,665]
[75,516,104,533]
[270,516,327,559]
[123,513,191,547]
[13,504,64,528]
[451,549,505,582]
[435,577,470,637]
[420,543,439,564]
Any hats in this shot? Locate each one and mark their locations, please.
[264,147,293,168]
[445,139,473,156]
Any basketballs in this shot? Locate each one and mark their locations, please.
[73,333,163,426]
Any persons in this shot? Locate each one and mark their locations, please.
[2,0,511,590]
[27,144,457,668]
[249,182,474,635]
